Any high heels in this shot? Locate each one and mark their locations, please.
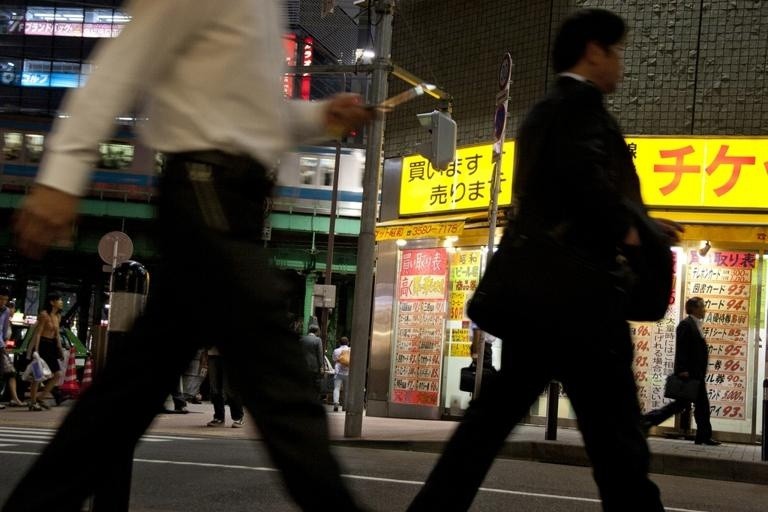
[1,397,48,411]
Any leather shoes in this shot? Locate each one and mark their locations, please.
[56,394,70,405]
[695,439,722,445]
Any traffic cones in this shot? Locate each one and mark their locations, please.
[81,353,93,392]
[58,348,79,394]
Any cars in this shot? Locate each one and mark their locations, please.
[4,322,89,382]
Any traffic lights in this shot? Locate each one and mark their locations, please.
[412,112,459,171]
[340,123,364,145]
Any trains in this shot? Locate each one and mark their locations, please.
[0,105,383,226]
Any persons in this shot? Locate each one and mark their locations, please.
[407,7,686,512]
[639,296,721,446]
[157,345,249,427]
[0,0,385,511]
[1,288,64,411]
[299,325,351,412]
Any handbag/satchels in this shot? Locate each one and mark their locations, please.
[2,350,17,376]
[337,350,350,365]
[665,375,700,404]
[620,239,671,322]
[460,367,498,392]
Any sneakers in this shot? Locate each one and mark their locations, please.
[208,419,225,426]
[232,417,247,428]
